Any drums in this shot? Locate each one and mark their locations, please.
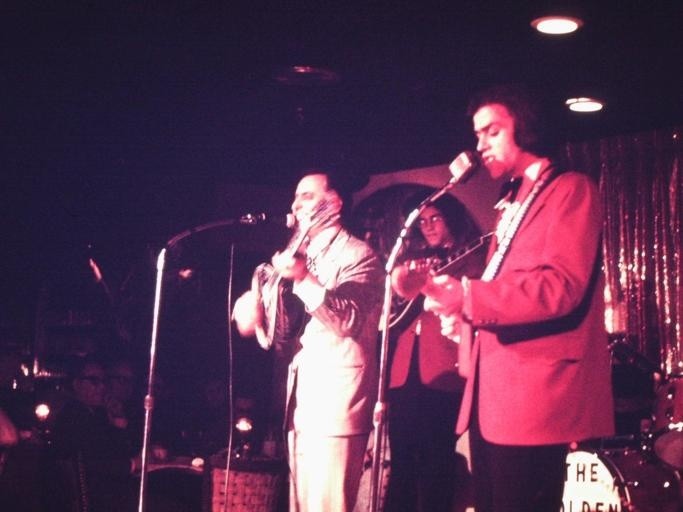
[558,445,682,511]
[640,376,683,470]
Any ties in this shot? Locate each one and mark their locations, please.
[493,175,521,208]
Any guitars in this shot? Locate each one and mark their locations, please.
[392,260,478,376]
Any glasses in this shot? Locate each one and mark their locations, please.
[415,215,444,229]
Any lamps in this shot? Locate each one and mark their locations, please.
[564,96,607,115]
[528,11,587,35]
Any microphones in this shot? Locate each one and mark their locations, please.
[240,212,295,229]
[447,149,480,184]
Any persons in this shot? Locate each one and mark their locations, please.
[423,82,616,511]
[361,188,492,511]
[231,170,385,511]
